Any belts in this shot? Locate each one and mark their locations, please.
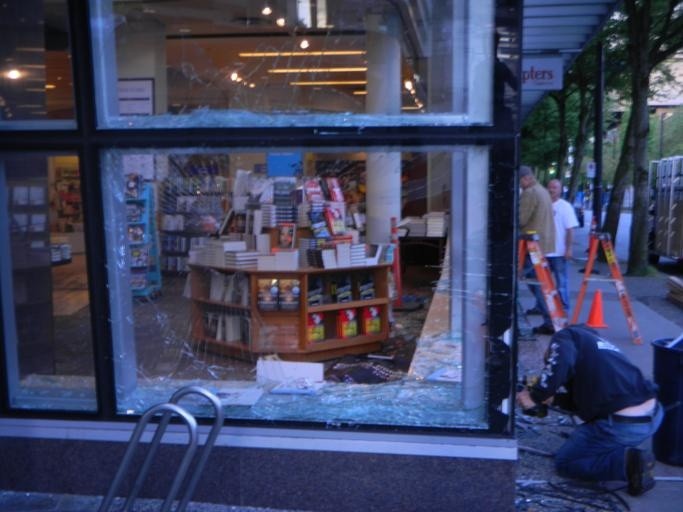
[600,400,660,424]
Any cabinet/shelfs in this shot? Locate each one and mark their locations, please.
[165,190,229,274]
[193,265,392,362]
[126,181,162,296]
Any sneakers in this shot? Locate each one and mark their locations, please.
[627,448,655,496]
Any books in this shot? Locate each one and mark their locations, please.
[398,212,449,237]
[162,176,394,343]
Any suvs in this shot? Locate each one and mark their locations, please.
[562,188,584,228]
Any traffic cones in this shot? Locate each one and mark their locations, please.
[586,287,609,329]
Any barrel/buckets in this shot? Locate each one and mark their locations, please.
[649,336,679,464]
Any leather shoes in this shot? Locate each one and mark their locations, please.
[533,323,553,334]
[527,307,543,315]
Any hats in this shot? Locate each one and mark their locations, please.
[518,164,531,178]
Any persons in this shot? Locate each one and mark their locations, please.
[519,166,555,334]
[526,178,580,316]
[515,323,665,495]
[563,180,607,227]
